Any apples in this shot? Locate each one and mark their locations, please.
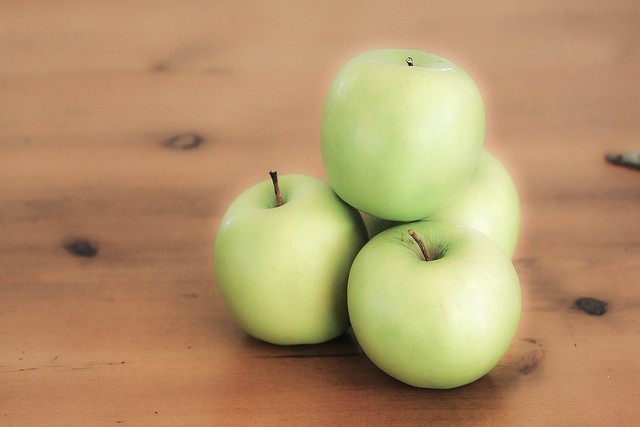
[434,148,519,261]
[319,46,484,221]
[347,221,521,390]
[213,169,370,347]
